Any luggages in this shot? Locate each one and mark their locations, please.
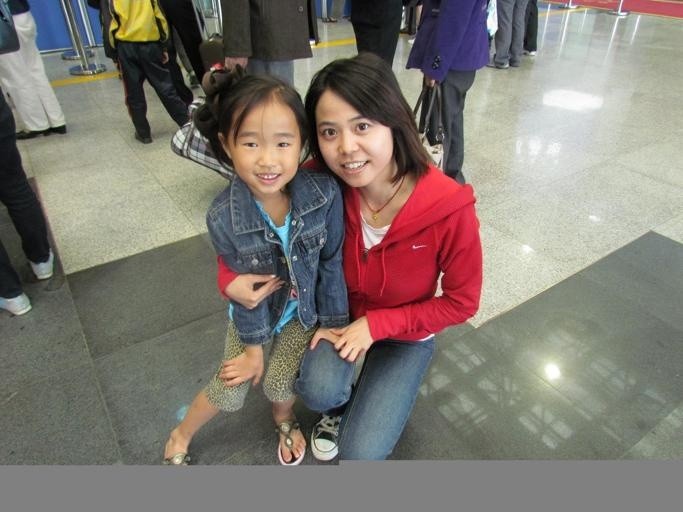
[191,0,224,72]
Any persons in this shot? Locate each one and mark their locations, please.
[487,0,537,69]
[87,0,491,185]
[0,94,54,315]
[161,76,348,466]
[215,52,485,463]
[0,0,67,140]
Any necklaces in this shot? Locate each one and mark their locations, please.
[359,173,404,220]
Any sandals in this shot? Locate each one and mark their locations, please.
[164,440,191,466]
[273,420,305,466]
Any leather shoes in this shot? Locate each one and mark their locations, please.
[50,123,66,134]
[135,132,151,143]
[14,126,50,139]
[400,26,409,34]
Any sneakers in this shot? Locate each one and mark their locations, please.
[310,413,342,461]
[1,293,32,316]
[29,247,54,280]
[523,49,537,56]
[487,55,509,69]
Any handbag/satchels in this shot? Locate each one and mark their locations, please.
[413,83,445,171]
[171,62,247,181]
